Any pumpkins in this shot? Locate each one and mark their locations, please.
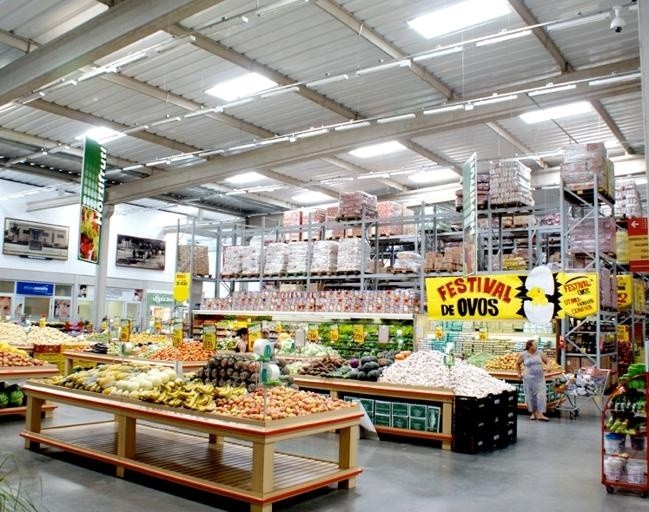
[343,356,393,380]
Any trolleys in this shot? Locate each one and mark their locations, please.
[555,369,611,420]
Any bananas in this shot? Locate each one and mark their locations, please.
[139,374,248,412]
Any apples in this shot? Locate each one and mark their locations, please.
[0,350,48,366]
[216,386,358,420]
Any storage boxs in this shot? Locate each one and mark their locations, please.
[518,391,561,404]
[344,396,442,433]
[566,357,618,390]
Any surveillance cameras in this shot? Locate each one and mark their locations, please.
[610,17,626,33]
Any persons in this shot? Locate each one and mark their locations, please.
[515,339,551,421]
[252,327,278,360]
[131,240,165,260]
[582,364,606,397]
[15,302,22,320]
[235,327,248,352]
[134,289,143,301]
[276,328,291,345]
[78,285,87,297]
[55,301,71,319]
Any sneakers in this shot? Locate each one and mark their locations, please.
[528,415,551,422]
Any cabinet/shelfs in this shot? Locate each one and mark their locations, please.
[601,371,649,498]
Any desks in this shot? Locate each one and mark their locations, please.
[489,370,567,410]
[292,376,457,450]
[18,379,364,512]
[17,346,36,353]
[0,365,60,420]
[61,349,205,377]
